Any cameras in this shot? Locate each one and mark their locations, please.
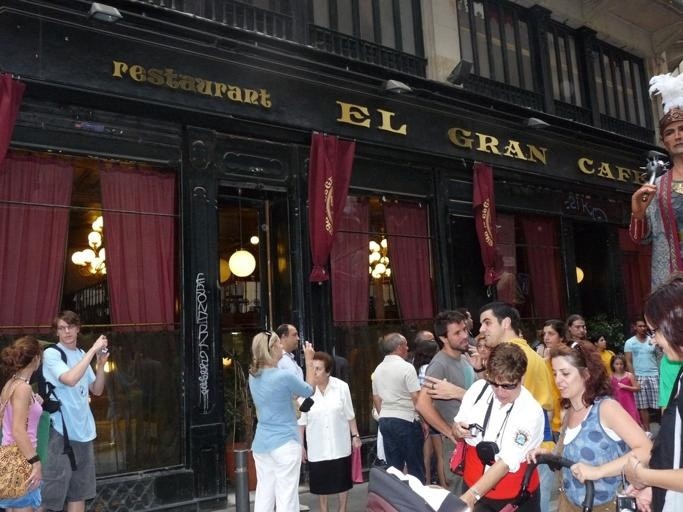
[462,424,484,438]
[98,346,108,357]
[616,495,637,512]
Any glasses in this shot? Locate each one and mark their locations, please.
[562,339,581,350]
[485,378,520,390]
[645,328,658,337]
[265,330,271,353]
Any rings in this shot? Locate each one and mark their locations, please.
[431,384,435,389]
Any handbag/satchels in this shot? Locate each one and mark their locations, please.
[37,343,67,413]
[0,382,33,500]
[555,412,617,512]
[449,383,489,476]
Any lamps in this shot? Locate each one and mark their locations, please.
[250,235,260,246]
[228,248,256,277]
[365,236,395,284]
[71,212,109,278]
[576,267,584,283]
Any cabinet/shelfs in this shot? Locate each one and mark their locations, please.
[65,279,113,322]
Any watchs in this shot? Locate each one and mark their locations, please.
[352,434,360,438]
[469,488,481,502]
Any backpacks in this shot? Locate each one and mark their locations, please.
[37,343,83,415]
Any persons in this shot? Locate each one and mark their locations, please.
[413,340,448,488]
[525,339,653,512]
[251,330,315,512]
[626,74,683,297]
[544,320,569,348]
[298,352,362,511]
[0,336,43,512]
[609,355,641,427]
[623,274,683,512]
[411,331,435,364]
[566,314,586,339]
[456,308,474,329]
[277,324,305,381]
[415,311,478,498]
[452,342,545,512]
[371,332,426,486]
[421,301,554,452]
[624,318,661,432]
[43,310,110,512]
[590,333,616,374]
[473,334,490,367]
[413,309,474,383]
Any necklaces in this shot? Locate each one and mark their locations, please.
[570,399,587,412]
